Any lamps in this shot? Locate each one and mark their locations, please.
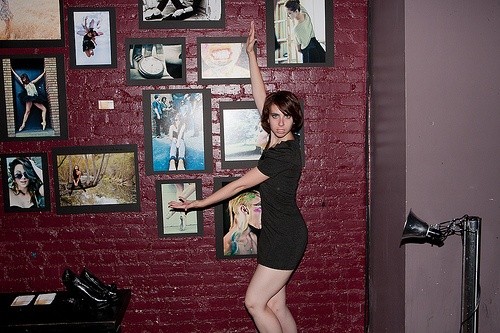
[398,208,464,247]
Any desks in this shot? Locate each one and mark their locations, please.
[0,289,131,333]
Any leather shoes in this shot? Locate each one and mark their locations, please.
[145,13,162,20]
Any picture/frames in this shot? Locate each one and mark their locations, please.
[155,179,203,238]
[0,54,69,141]
[265,0,334,68]
[0,153,51,213]
[68,7,118,70]
[125,37,186,86]
[139,0,225,30]
[52,145,141,214]
[219,102,304,168]
[142,88,214,175]
[196,36,257,84]
[137,0,225,30]
[0,0,65,49]
[214,177,262,260]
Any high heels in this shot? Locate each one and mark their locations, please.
[79,268,121,302]
[62,269,112,310]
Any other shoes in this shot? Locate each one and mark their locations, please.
[166,209,175,219]
[178,214,186,231]
[41,122,47,131]
[19,125,24,131]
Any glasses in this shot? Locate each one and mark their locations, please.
[14,170,30,179]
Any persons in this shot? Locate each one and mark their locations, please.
[80,17,104,57]
[167,20,310,332]
[6,158,44,208]
[284,0,326,64]
[151,95,203,171]
[164,184,196,229]
[201,43,251,79]
[68,165,87,195]
[145,0,202,20]
[10,66,48,131]
